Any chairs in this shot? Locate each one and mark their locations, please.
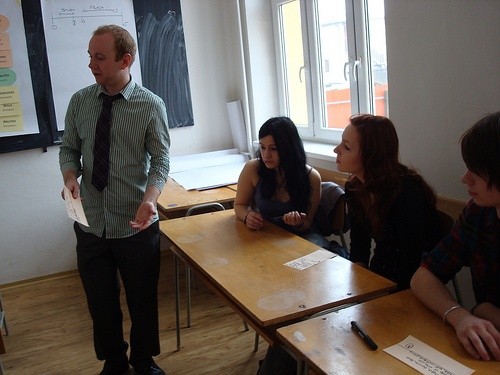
[314,182,350,259]
[183,202,226,327]
[436,210,468,309]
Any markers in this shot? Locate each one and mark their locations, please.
[351,321,378,350]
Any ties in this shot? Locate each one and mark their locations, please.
[91,93,125,192]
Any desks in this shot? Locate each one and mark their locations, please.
[155,178,500,375]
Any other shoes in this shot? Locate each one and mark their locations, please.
[130,356,166,373]
[98,357,130,375]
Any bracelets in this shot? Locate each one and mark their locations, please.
[443,305,464,330]
[244,212,249,225]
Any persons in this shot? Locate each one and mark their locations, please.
[59,24,171,375]
[411,112,500,362]
[235,116,321,229]
[256,114,438,375]
